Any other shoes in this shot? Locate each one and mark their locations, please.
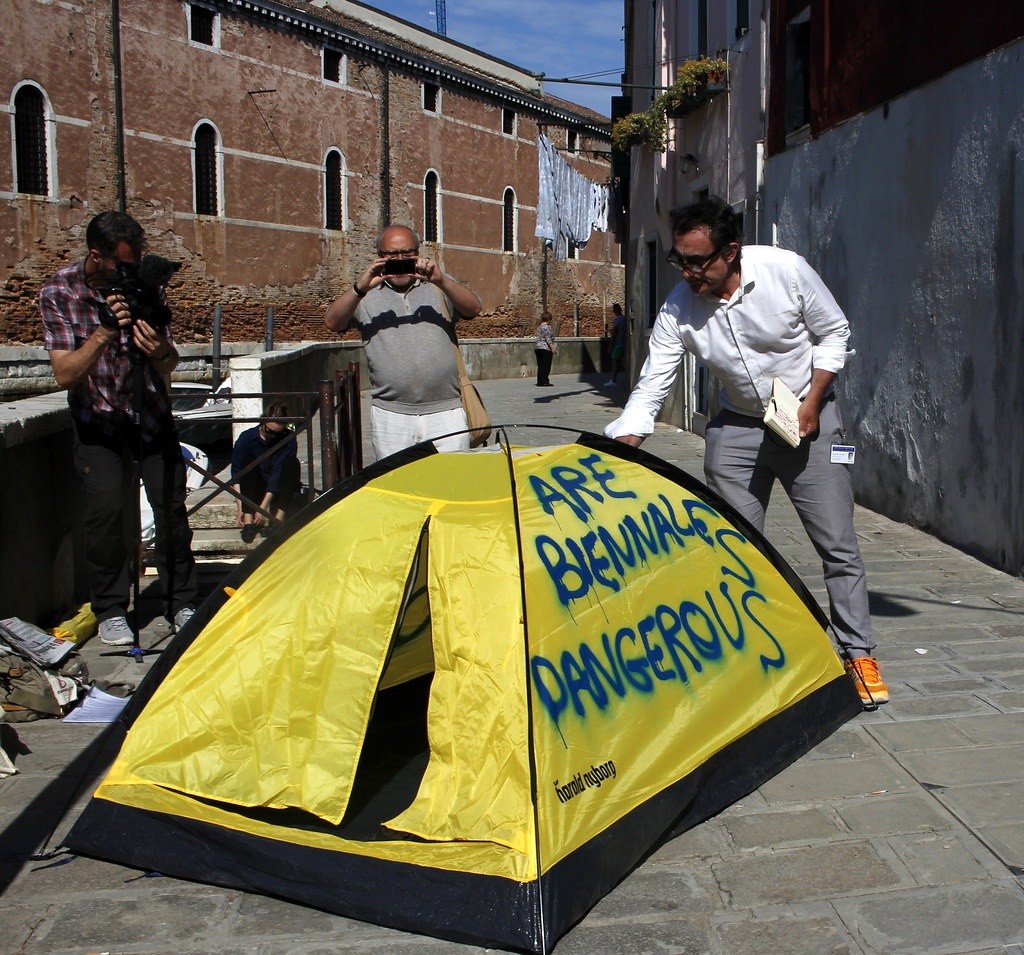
[260,522,280,537]
[537,383,553,386]
[240,525,256,543]
[603,379,618,387]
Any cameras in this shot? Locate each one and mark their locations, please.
[384,258,417,274]
[99,260,173,330]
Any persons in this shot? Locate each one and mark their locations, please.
[604,196,889,703]
[534,311,556,387]
[232,401,301,543]
[40,210,197,644]
[323,225,482,461]
[603,304,626,386]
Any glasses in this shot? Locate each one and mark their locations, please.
[264,422,285,435]
[378,249,419,259]
[666,246,723,273]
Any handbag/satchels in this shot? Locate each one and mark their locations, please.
[459,377,491,447]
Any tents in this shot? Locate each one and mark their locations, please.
[69,424,879,955]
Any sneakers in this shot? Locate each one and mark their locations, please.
[171,607,196,634]
[98,617,135,646]
[844,658,890,704]
